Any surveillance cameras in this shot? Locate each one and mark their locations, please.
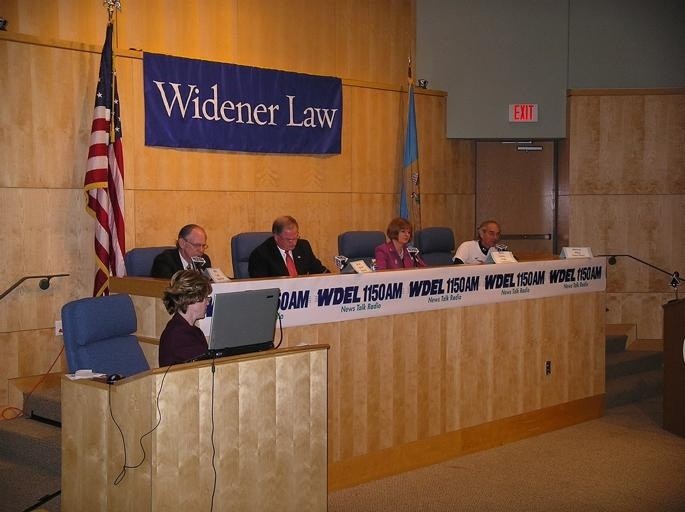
[418,79,428,88]
[0,18,7,30]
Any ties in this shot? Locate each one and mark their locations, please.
[285,250,298,278]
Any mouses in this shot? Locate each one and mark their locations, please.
[109,374,120,381]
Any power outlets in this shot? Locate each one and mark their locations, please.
[55,321,63,335]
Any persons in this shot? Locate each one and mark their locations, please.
[373,216,427,269]
[156,268,213,367]
[451,220,508,264]
[248,214,331,277]
[149,224,210,282]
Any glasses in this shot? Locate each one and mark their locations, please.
[183,239,208,251]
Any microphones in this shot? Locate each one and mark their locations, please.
[406,243,420,263]
[191,256,206,274]
[333,255,349,270]
[668,271,681,291]
[495,244,508,252]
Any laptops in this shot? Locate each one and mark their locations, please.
[207,288,280,359]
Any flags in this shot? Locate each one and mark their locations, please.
[82,21,128,296]
[399,62,422,244]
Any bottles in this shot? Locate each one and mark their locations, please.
[370,258,377,272]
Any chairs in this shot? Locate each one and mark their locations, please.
[126,246,182,277]
[230,232,276,279]
[336,230,389,273]
[415,226,454,267]
[59,293,149,375]
